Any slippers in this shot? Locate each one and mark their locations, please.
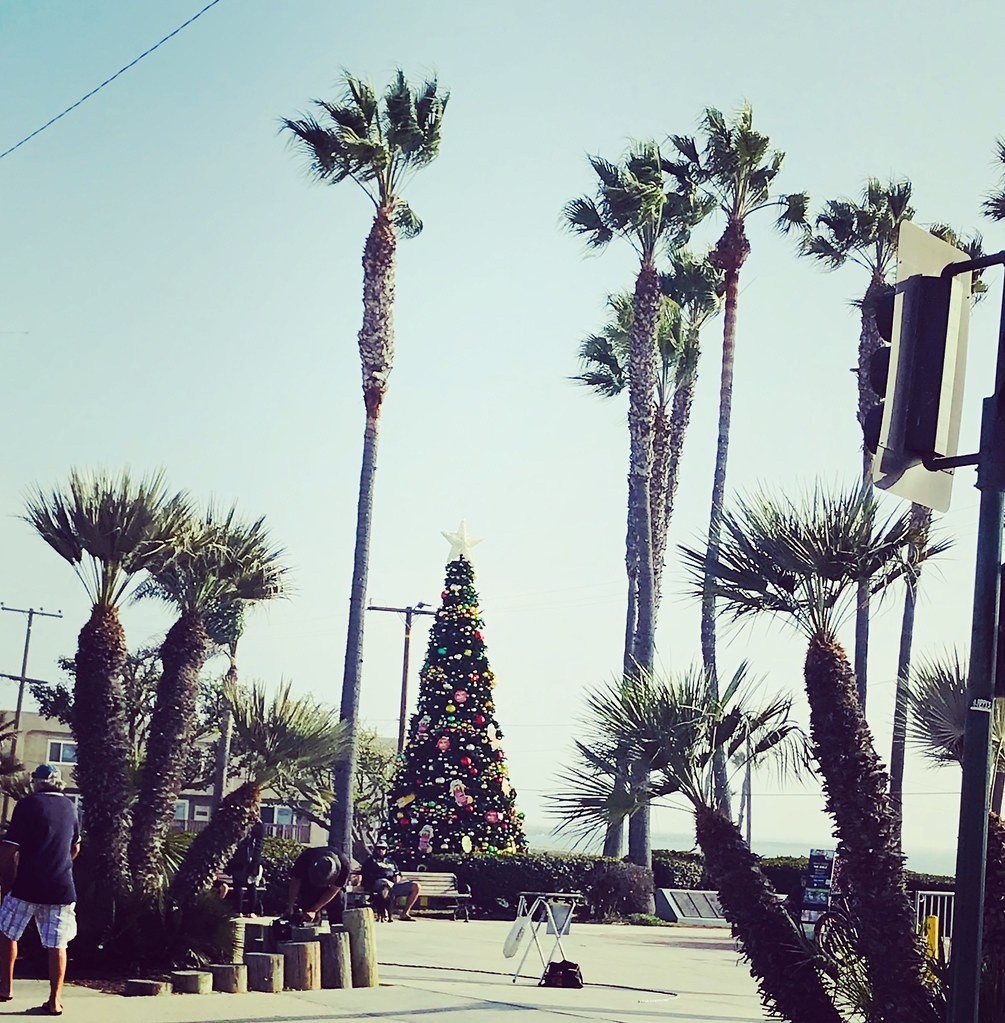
[41,1002,62,1017]
[398,915,417,922]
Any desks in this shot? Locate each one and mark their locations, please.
[503,891,583,984]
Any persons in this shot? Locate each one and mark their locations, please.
[229,822,263,917]
[361,840,421,922]
[0,765,82,1015]
[209,868,228,899]
[281,846,351,926]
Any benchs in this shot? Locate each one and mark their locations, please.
[348,870,472,924]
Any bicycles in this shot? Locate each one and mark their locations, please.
[814,880,869,967]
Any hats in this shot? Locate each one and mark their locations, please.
[375,839,389,848]
[30,763,59,781]
